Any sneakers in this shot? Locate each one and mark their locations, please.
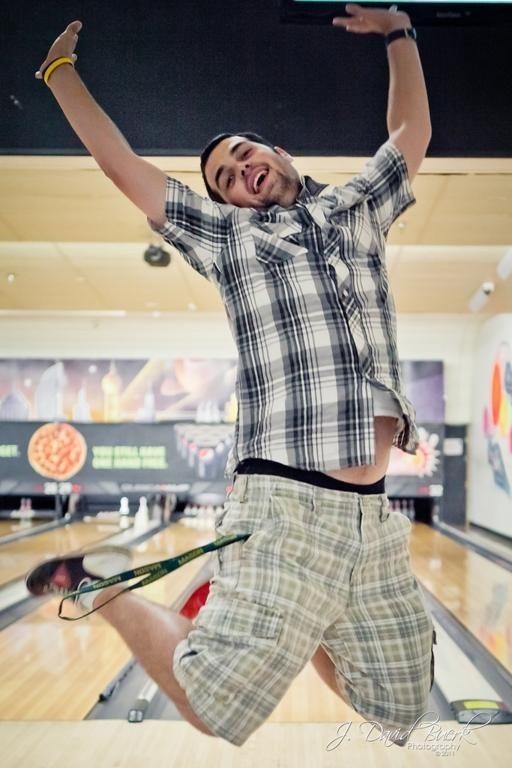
[24,542,135,605]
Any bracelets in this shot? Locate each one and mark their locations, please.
[42,56,74,83]
[384,27,418,47]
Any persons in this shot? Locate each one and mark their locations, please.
[26,5,432,746]
